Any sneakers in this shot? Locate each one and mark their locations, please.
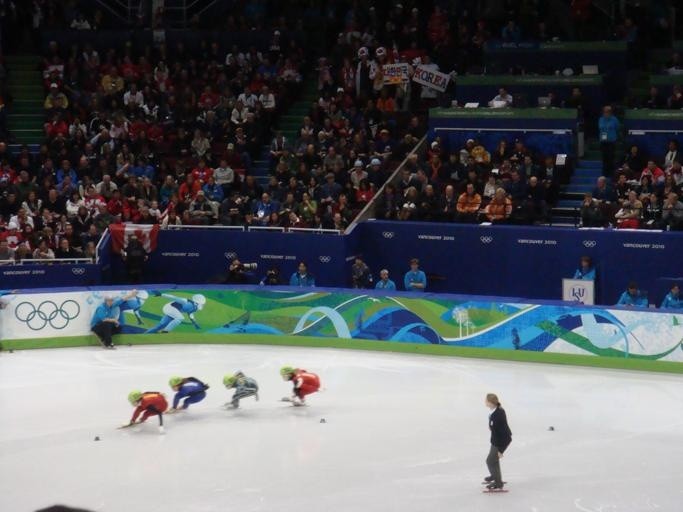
[487,482,503,488]
[485,476,494,481]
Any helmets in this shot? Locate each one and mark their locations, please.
[193,294,205,304]
[223,374,237,385]
[104,293,113,301]
[137,290,148,299]
[170,377,183,386]
[280,366,294,374]
[127,391,142,401]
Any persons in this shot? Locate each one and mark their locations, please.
[164,373,209,414]
[279,364,321,407]
[125,391,169,434]
[221,369,259,410]
[87,288,139,347]
[482,394,513,487]
[144,293,207,337]
[1,1,682,312]
